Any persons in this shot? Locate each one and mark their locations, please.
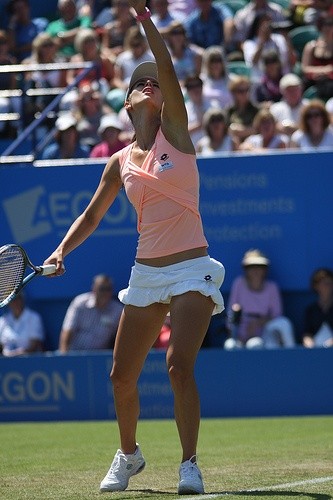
[43,0,225,494]
[0,0,333,158]
[0,250,333,356]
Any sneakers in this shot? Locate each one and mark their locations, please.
[100,442,145,493]
[177,455,205,494]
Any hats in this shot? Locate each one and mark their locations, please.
[97,112,124,138]
[240,249,268,266]
[278,73,302,93]
[125,61,159,103]
[55,112,77,132]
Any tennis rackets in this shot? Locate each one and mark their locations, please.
[0,243,65,309]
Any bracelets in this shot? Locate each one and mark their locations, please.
[136,7,151,21]
